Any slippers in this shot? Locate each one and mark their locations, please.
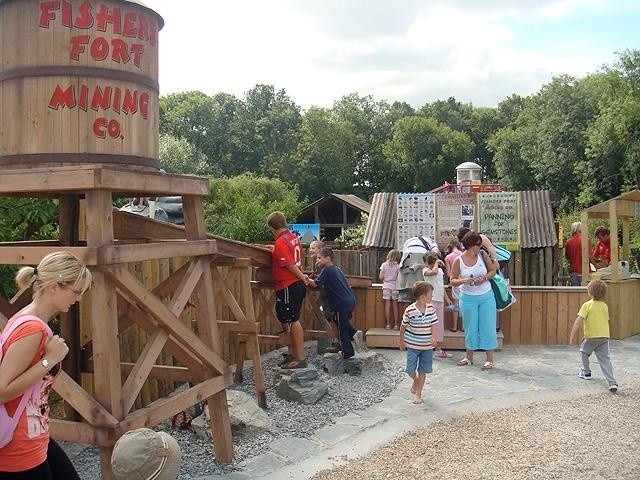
[481,361,497,370]
[457,358,473,366]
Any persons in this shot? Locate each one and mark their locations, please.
[265,211,310,370]
[399,280,439,404]
[590,226,610,269]
[378,227,500,370]
[569,279,618,392]
[309,240,325,274]
[0,250,94,479]
[308,247,363,360]
[564,221,592,286]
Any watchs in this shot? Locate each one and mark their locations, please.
[41,355,51,372]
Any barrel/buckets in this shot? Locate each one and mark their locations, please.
[0,0,165,175]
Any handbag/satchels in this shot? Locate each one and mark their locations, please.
[491,274,516,312]
[488,242,513,268]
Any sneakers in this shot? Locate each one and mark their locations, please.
[434,350,452,358]
[279,353,307,369]
[577,371,592,380]
[609,385,618,391]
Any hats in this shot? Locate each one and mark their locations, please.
[111,428,182,480]
